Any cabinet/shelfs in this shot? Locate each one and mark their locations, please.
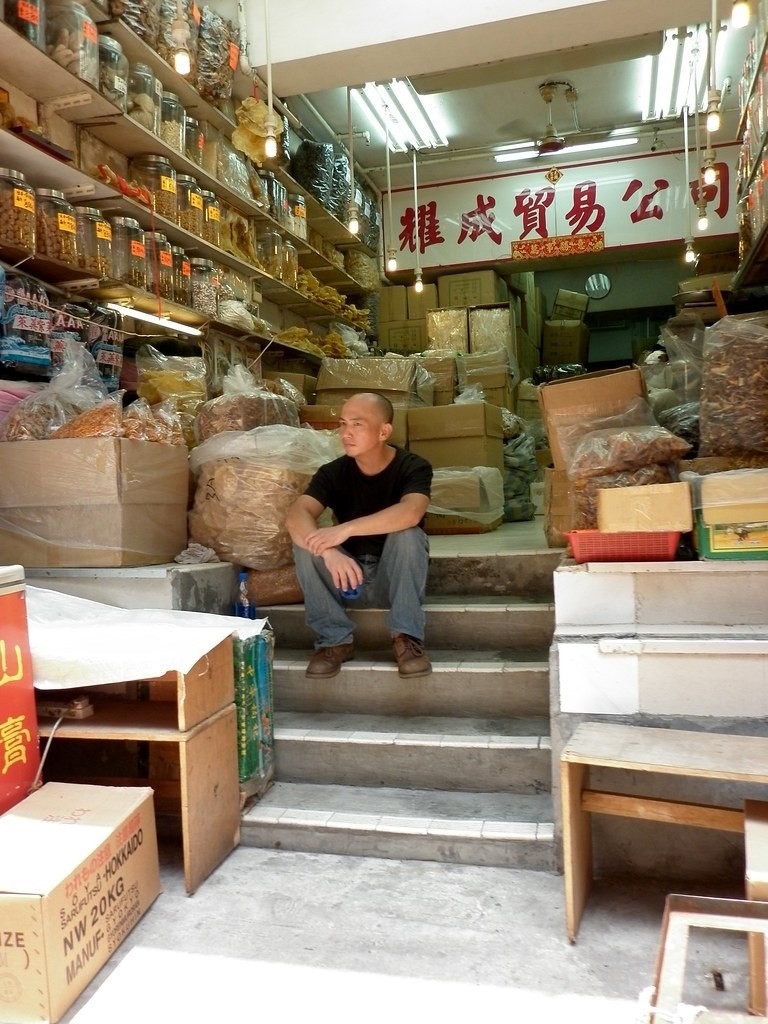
[732,38,768,287]
[0,0,382,365]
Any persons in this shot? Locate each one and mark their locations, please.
[285,392,433,680]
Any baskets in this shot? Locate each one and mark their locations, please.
[565,530,681,564]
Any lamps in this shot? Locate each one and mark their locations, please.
[706,0,720,132]
[681,106,695,263]
[692,49,708,231]
[411,146,423,293]
[382,104,397,271]
[704,132,717,186]
[348,76,449,154]
[172,1,191,74]
[104,302,205,336]
[263,1,277,158]
[641,18,727,121]
[347,85,359,234]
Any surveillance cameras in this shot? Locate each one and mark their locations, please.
[650,142,663,152]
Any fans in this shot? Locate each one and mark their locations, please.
[500,83,611,154]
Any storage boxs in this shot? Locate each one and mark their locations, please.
[218,96,236,126]
[262,271,590,535]
[231,98,241,111]
[0,437,189,567]
[539,362,647,470]
[674,455,768,562]
[0,782,163,1023]
[209,335,261,386]
[544,467,574,548]
[633,271,737,361]
[232,629,275,816]
[595,482,693,532]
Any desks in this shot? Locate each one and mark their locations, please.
[559,721,768,945]
[39,698,241,895]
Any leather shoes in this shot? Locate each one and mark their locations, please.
[305,644,355,678]
[393,633,432,678]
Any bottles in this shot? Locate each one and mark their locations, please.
[108,217,145,287]
[76,207,112,276]
[191,258,217,317]
[256,222,283,279]
[145,231,171,299]
[235,573,256,621]
[1,1,305,232]
[289,194,307,241]
[281,241,298,289]
[0,167,37,254]
[172,246,192,305]
[32,188,75,263]
